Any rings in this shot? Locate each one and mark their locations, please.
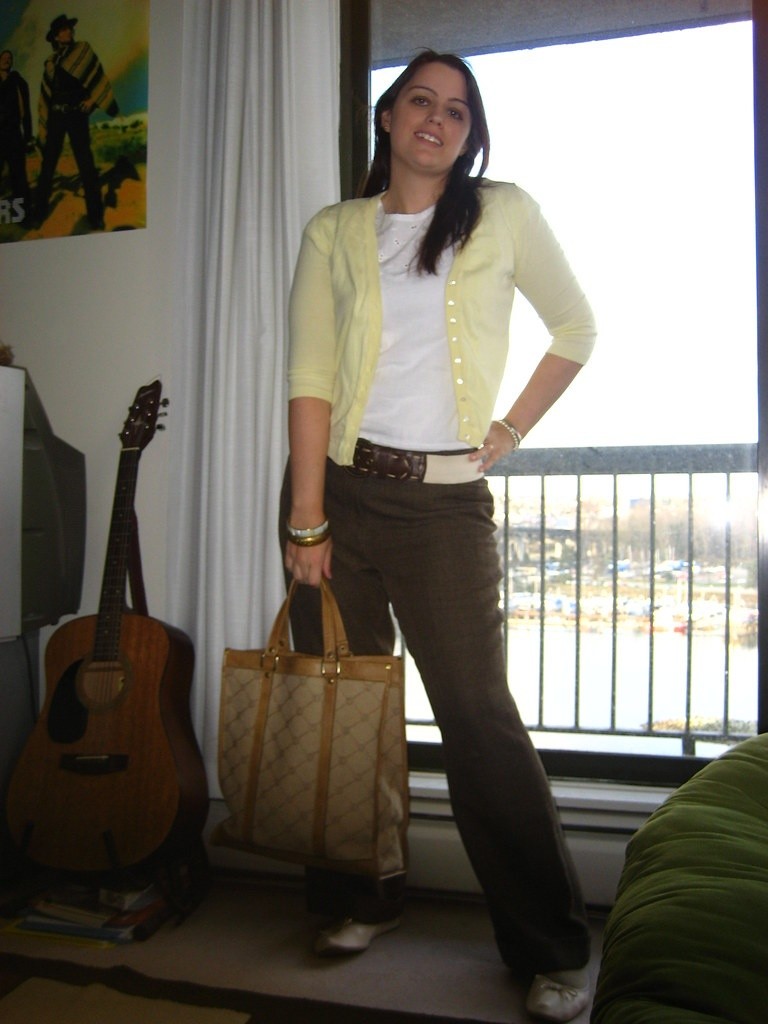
[489,442,494,449]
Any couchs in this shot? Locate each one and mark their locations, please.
[590,733,768,1024]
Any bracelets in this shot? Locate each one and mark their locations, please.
[285,528,332,546]
[494,419,521,449]
[282,519,331,537]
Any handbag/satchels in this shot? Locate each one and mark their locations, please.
[210,577,411,883]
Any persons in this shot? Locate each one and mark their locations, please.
[0,13,118,229]
[278,48,602,1024]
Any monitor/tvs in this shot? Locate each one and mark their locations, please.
[0,365,87,643]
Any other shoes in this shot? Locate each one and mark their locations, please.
[525,974,590,1021]
[316,916,402,951]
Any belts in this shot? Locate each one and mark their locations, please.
[342,437,485,484]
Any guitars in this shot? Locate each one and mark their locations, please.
[4,381,211,890]
